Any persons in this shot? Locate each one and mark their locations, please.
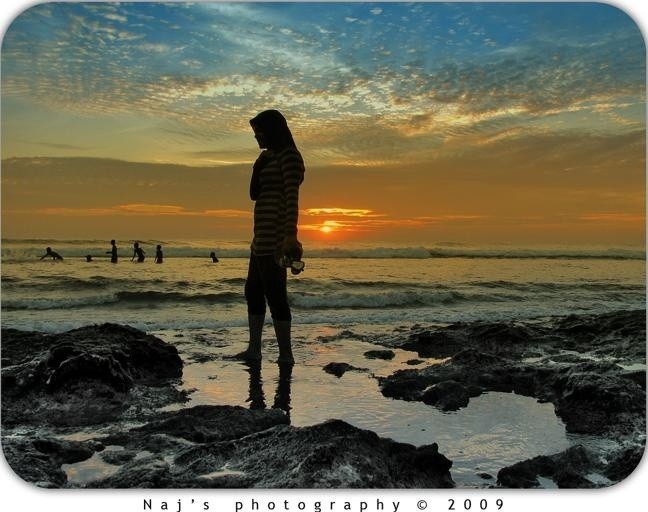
[131,242,145,262]
[153,245,163,263]
[85,255,93,261]
[106,239,117,263]
[210,252,219,263]
[222,109,305,364]
[40,248,65,261]
